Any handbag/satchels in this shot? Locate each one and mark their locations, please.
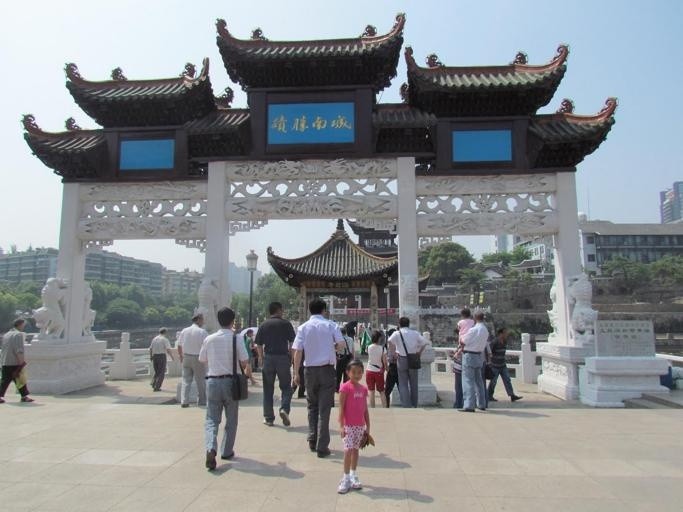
[407,353,420,369]
[232,374,248,400]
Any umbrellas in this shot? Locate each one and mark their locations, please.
[241,327,259,336]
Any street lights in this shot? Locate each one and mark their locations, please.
[243,249,259,327]
[383,284,390,329]
[354,295,359,320]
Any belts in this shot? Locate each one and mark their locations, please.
[205,375,232,379]
[463,351,481,354]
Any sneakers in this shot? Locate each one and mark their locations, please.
[280,409,290,425]
[337,479,362,493]
[264,419,273,425]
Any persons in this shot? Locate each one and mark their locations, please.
[199,306,249,469]
[177,313,208,407]
[149,327,174,391]
[291,297,346,458]
[291,349,306,398]
[337,358,370,492]
[335,317,429,408]
[449,307,524,412]
[254,302,296,426]
[0,318,35,402]
[244,329,257,378]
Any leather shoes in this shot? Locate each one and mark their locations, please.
[511,395,522,401]
[206,449,216,468]
[221,451,234,459]
